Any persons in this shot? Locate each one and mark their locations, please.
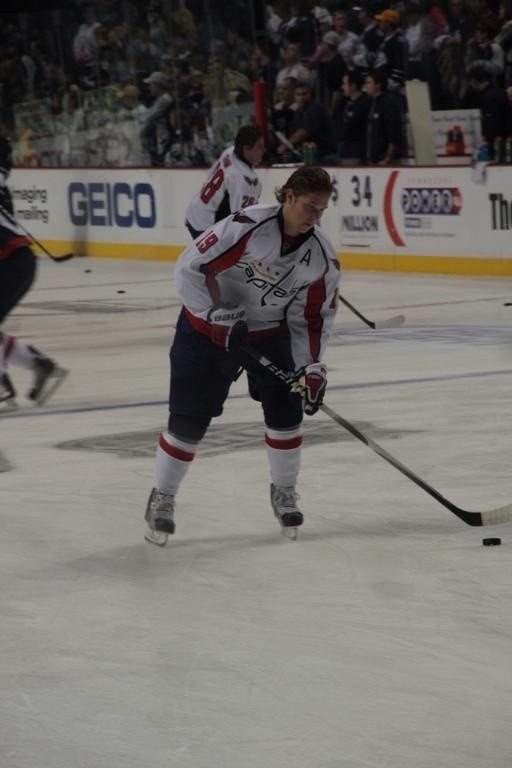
[185,127,266,241]
[145,167,341,534]
[0,0,511,169]
[1,172,57,403]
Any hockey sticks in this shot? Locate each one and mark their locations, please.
[0,207,75,263]
[337,293,404,330]
[242,342,512,526]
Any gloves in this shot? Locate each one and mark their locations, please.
[209,303,248,348]
[304,373,326,416]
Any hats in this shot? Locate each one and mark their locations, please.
[322,31,340,45]
[375,9,400,23]
[141,72,170,85]
[371,70,392,86]
[115,85,140,98]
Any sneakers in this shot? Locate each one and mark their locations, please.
[27,344,54,399]
[144,487,175,534]
[0,374,15,402]
[271,483,303,527]
